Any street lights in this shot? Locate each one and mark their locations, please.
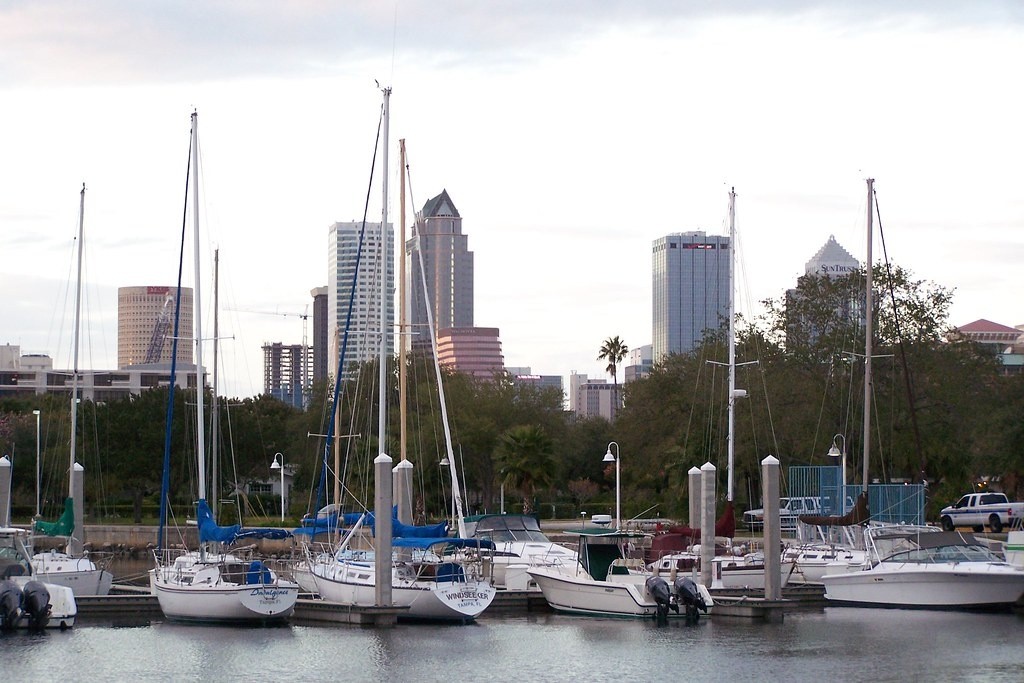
[828,433,845,519]
[601,441,621,532]
[270,452,284,524]
[440,449,456,528]
[31,411,41,520]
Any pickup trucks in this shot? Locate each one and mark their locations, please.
[742,497,856,531]
[941,492,1024,533]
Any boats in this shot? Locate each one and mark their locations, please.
[443,510,581,583]
[817,520,1024,613]
[1,528,79,630]
[528,526,714,621]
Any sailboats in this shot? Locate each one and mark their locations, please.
[25,83,942,626]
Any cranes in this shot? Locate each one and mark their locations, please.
[221,301,313,408]
